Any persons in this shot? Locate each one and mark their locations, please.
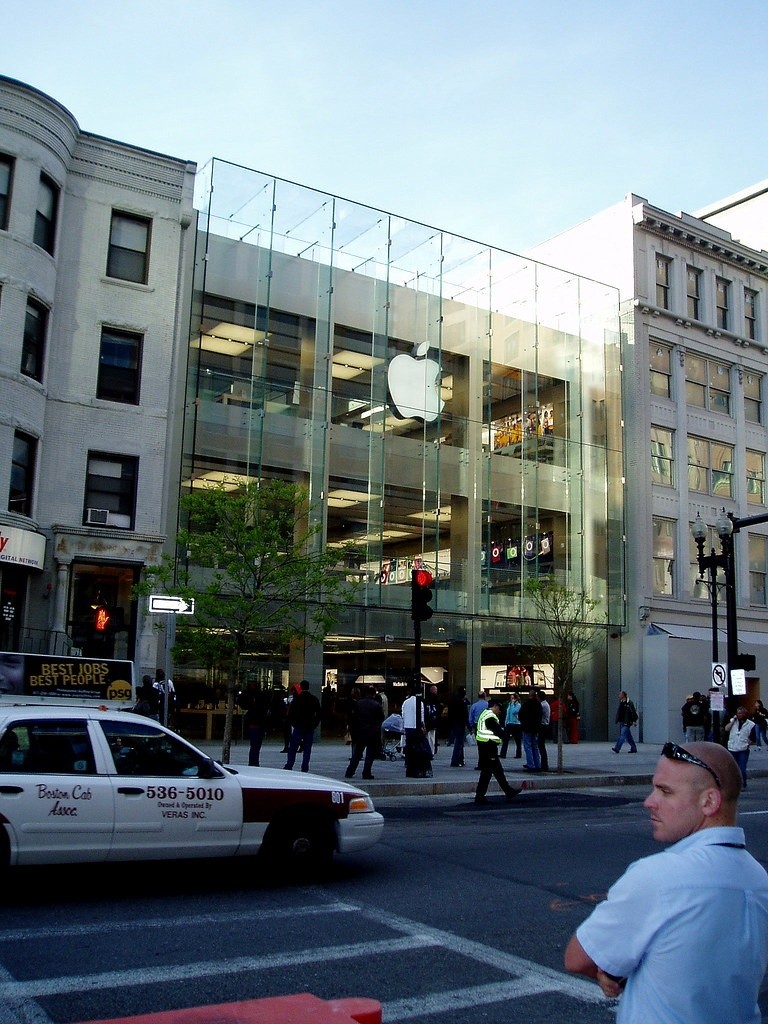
[725,706,756,792]
[565,741,768,1024]
[0,655,24,693]
[518,690,550,770]
[344,686,385,778]
[474,699,522,806]
[322,686,388,737]
[528,410,553,436]
[401,687,444,767]
[284,681,321,772]
[550,693,580,744]
[612,691,638,753]
[135,669,176,728]
[749,700,768,752]
[682,692,713,742]
[237,680,301,767]
[447,687,488,767]
[498,693,522,759]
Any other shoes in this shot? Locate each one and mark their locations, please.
[345,774,352,778]
[363,775,374,779]
[507,787,522,801]
[475,799,494,806]
[628,750,637,753]
[754,746,763,752]
[280,748,289,753]
[296,746,303,753]
[741,787,746,792]
[612,747,619,754]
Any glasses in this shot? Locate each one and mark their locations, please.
[661,742,721,788]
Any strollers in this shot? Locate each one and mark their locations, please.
[381,714,406,762]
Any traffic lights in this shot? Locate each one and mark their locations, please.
[410,570,434,622]
[95,606,124,633]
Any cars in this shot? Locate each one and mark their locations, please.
[0,704,383,887]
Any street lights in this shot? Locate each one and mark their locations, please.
[691,507,734,744]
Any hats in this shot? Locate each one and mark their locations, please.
[693,692,702,698]
[686,695,693,700]
